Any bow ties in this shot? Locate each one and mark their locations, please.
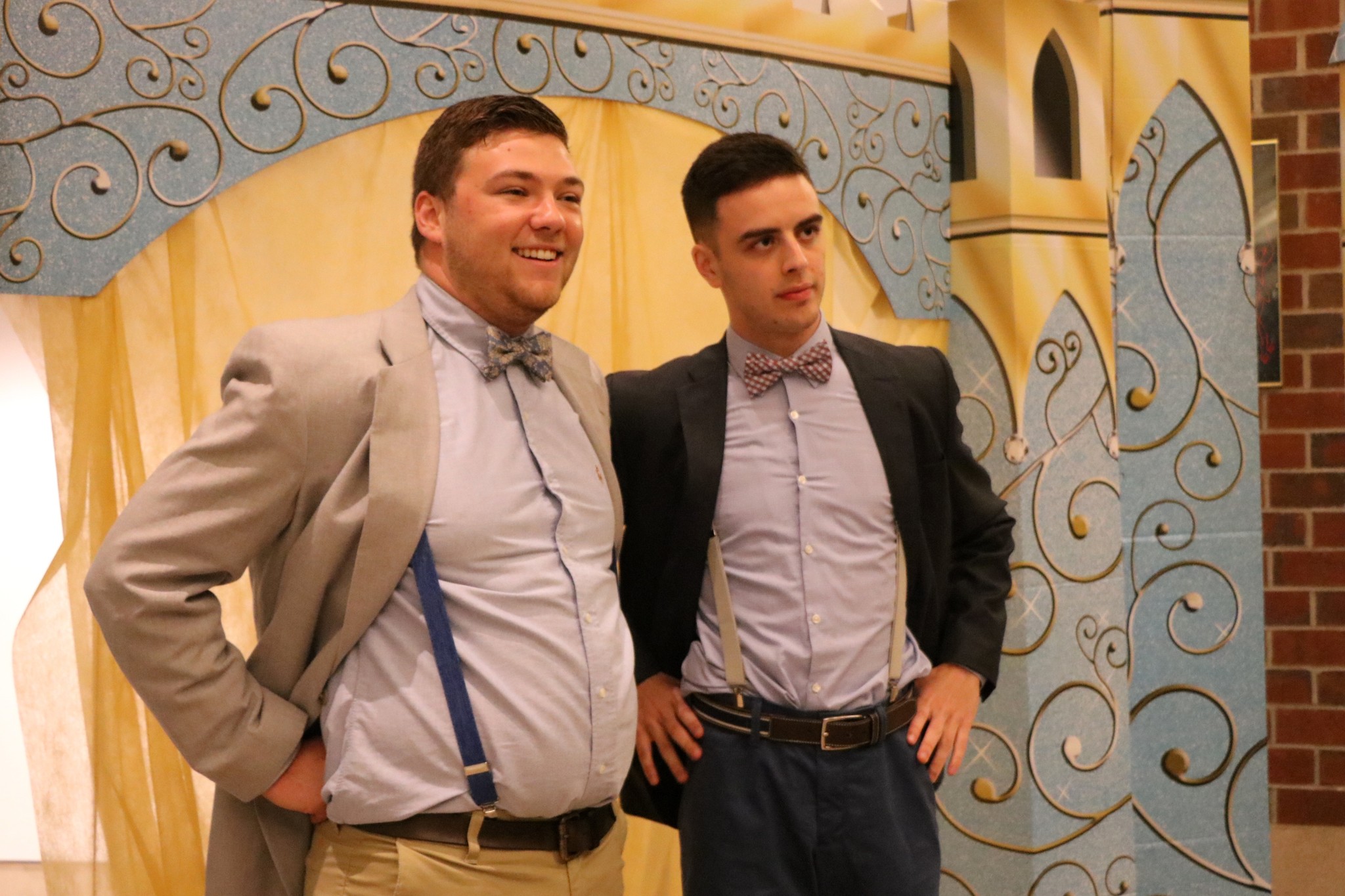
[743,339,832,399]
[480,324,554,383]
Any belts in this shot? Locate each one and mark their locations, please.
[689,684,918,750]
[343,798,616,863]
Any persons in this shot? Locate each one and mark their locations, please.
[82,93,635,896]
[604,134,1014,896]
